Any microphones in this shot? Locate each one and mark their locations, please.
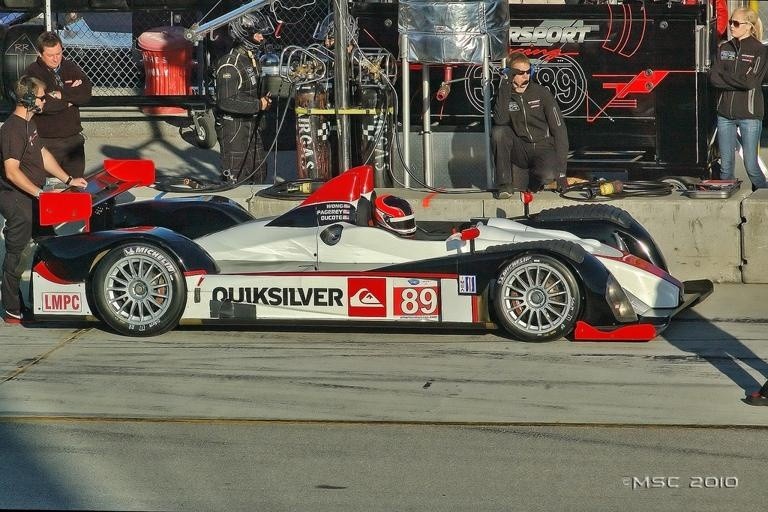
[513,80,528,88]
[27,108,41,113]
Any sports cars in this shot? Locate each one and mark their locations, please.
[30,159,715,342]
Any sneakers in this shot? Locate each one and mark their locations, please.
[4,309,39,323]
[497,184,513,199]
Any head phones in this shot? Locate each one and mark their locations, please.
[503,63,534,76]
[9,79,35,108]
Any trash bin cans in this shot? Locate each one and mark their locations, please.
[137,26,192,113]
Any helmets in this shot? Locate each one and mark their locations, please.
[374,194,416,235]
[313,12,358,44]
[230,11,275,52]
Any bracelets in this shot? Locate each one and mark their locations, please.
[64,175,73,186]
[33,189,45,198]
[51,90,56,98]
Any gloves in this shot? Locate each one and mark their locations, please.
[503,69,517,82]
[557,173,569,192]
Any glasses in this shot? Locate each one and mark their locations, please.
[36,95,45,100]
[518,68,530,75]
[729,19,745,27]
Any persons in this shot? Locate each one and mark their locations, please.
[314,12,390,178]
[491,51,569,199]
[216,10,272,184]
[744,379,768,406]
[710,8,766,188]
[1,74,88,324]
[23,32,92,176]
